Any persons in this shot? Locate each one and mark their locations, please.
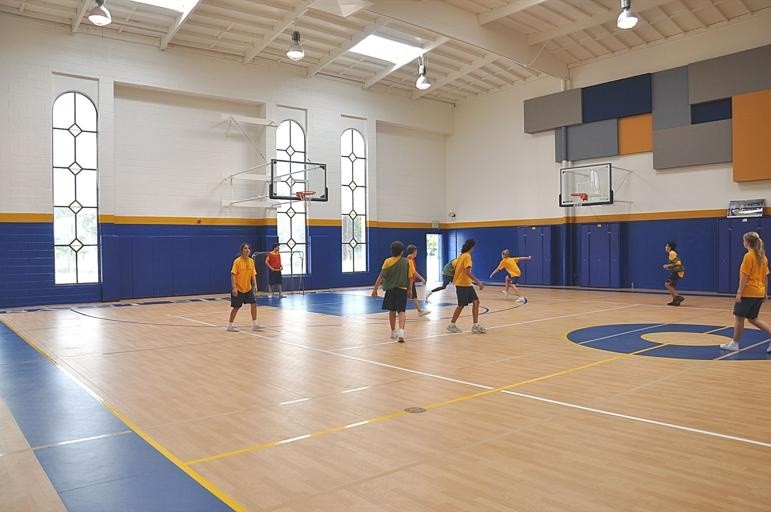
[425,258,457,300]
[720,231,771,353]
[407,244,431,317]
[265,242,287,298]
[371,241,413,342]
[490,250,531,302]
[226,242,265,332]
[663,241,685,306]
[446,239,489,334]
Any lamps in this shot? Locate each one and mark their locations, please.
[286,31,304,61]
[415,64,431,89]
[617,0,638,30]
[88,0,112,27]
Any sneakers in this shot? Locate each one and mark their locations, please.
[227,324,240,332]
[267,294,287,299]
[719,342,739,352]
[446,323,461,334]
[252,324,265,331]
[390,331,407,342]
[418,309,431,317]
[502,290,527,304]
[472,323,488,334]
[667,295,685,307]
